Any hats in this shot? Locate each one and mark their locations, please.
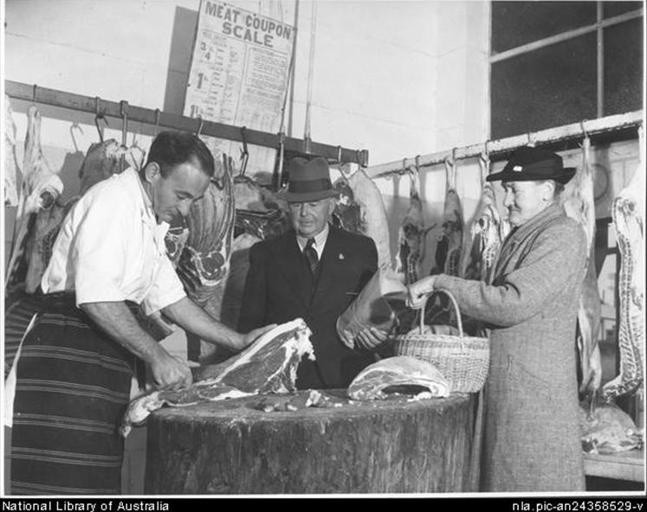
[276,154,341,204]
[486,146,576,184]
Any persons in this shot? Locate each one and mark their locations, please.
[4,131,278,497]
[403,147,587,490]
[235,154,394,392]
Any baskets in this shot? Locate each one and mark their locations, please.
[395,288,491,394]
